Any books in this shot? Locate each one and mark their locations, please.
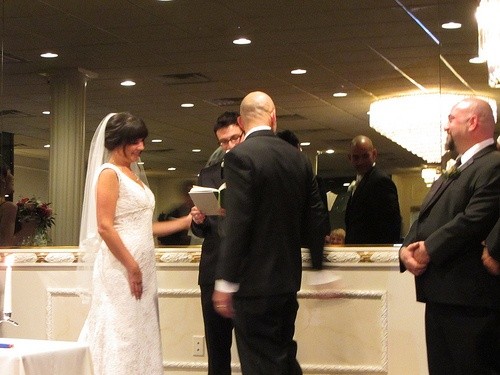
[188,181,226,215]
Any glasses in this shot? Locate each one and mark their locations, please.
[219,129,245,146]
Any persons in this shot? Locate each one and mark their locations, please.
[330,228,345,245]
[187,111,246,375]
[275,130,331,241]
[343,135,402,244]
[399,98,500,374]
[77,110,191,374]
[211,91,327,375]
[481,218,500,276]
[0,163,37,246]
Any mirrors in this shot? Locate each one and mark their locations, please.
[0,0,500,248]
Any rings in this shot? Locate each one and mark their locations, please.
[216,304,226,308]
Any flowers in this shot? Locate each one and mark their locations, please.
[16,198,56,234]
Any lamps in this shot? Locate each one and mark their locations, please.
[421,167,442,188]
[468,0,500,89]
[369,92,497,163]
